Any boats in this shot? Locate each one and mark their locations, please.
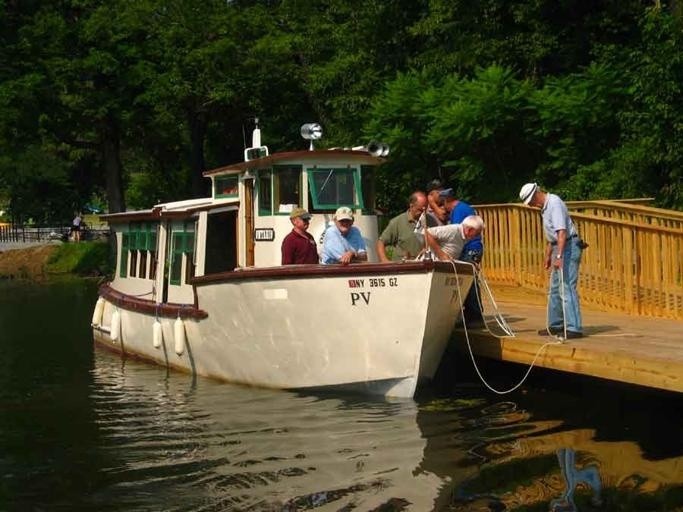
[88,116,481,404]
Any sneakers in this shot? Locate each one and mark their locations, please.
[557,330,584,340]
[538,327,564,334]
[289,207,313,220]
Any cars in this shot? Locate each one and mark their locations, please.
[81,206,105,214]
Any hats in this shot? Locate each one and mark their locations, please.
[426,179,449,192]
[519,182,538,206]
[438,187,456,199]
[334,206,353,222]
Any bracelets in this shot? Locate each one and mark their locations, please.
[554,254,563,259]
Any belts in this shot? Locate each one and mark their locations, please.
[551,233,580,246]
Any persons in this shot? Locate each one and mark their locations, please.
[374,191,429,263]
[420,214,483,261]
[70,216,83,244]
[416,179,448,226]
[438,188,483,328]
[319,206,368,264]
[519,183,584,340]
[280,208,319,264]
